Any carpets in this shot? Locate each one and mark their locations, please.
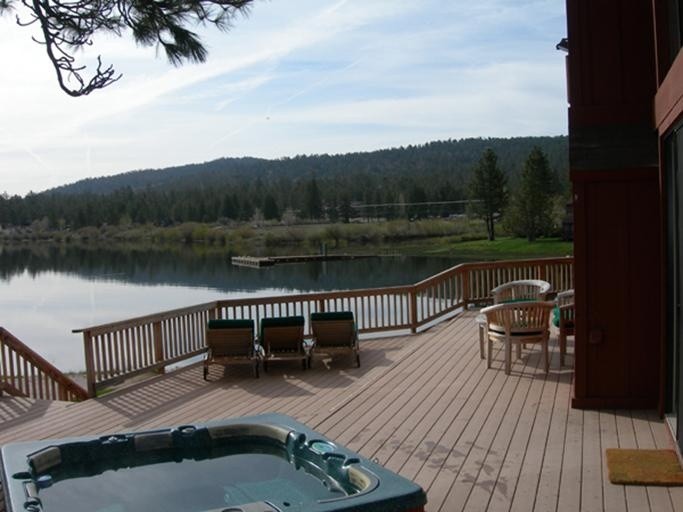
[606,448,682,487]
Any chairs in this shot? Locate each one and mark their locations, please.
[479,301,556,375]
[308,311,360,369]
[258,316,311,373]
[557,288,576,370]
[490,279,551,305]
[202,319,261,381]
